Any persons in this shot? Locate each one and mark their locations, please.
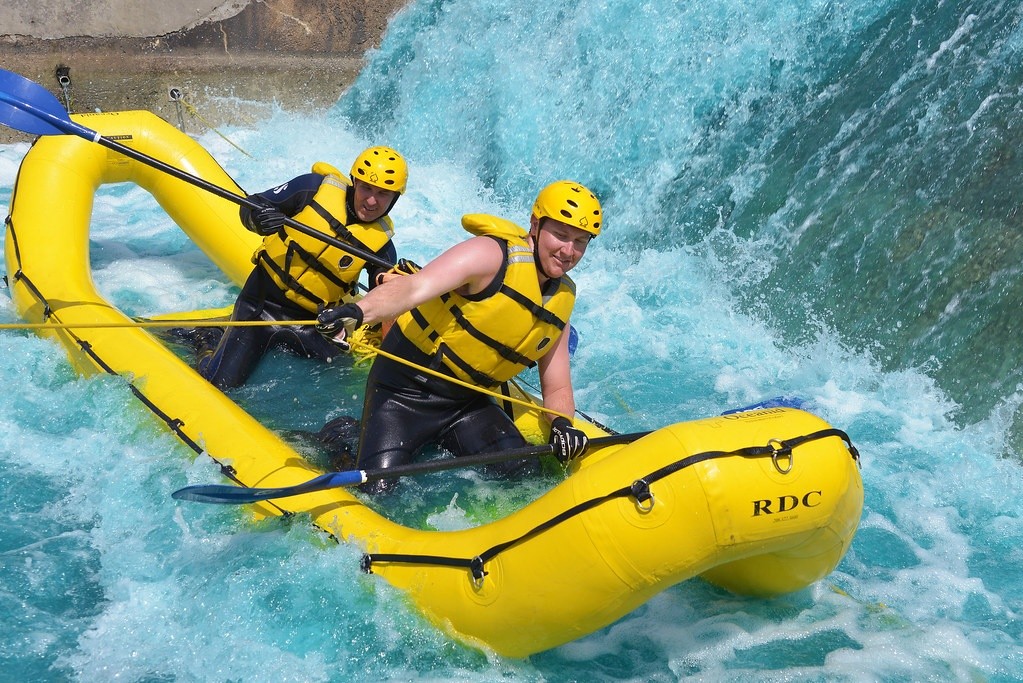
[315,180,603,490]
[168,147,408,387]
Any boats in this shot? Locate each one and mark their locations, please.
[4,109,865,660]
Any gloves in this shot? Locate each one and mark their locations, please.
[548,416,590,464]
[250,202,287,236]
[316,302,365,351]
[398,258,423,275]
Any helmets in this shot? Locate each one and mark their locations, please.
[349,145,409,196]
[531,179,603,235]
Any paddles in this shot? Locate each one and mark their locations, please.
[171,394,803,506]
[0,68,395,269]
